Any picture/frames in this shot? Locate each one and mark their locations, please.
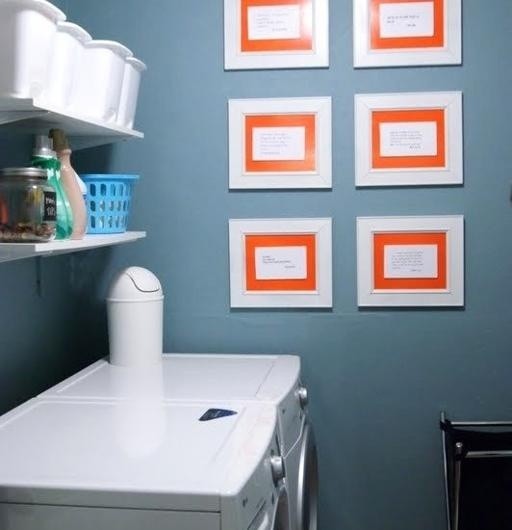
[353,0,461,68]
[228,96,332,189]
[224,0,330,71]
[352,91,463,186]
[356,215,464,306]
[228,217,333,308]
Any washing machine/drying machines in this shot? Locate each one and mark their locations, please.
[0,398,291,530]
[38,352,319,530]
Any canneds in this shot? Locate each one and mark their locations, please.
[0,168,56,243]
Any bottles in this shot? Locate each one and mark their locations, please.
[0,128,89,244]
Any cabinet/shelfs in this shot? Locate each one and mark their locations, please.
[0,92,147,263]
